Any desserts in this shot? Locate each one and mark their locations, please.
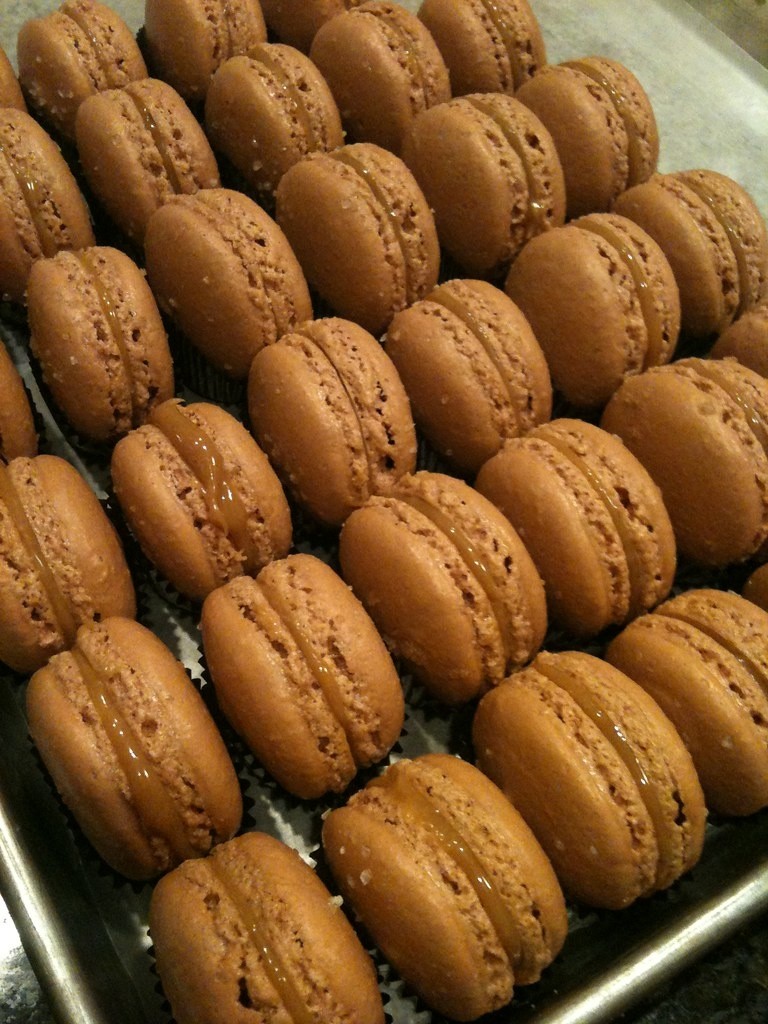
[0,0,768,1024]
